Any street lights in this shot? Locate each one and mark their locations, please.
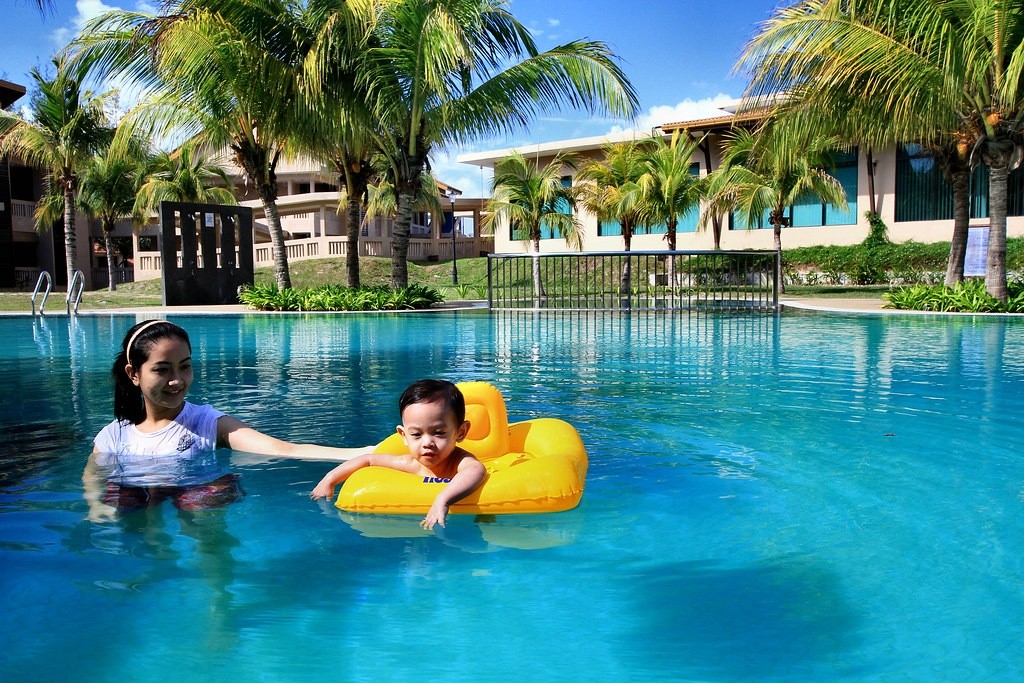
[447,191,458,285]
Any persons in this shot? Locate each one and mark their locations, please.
[89,317,377,460]
[309,379,488,525]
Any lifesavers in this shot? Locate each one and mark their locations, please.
[333,379,587,516]
